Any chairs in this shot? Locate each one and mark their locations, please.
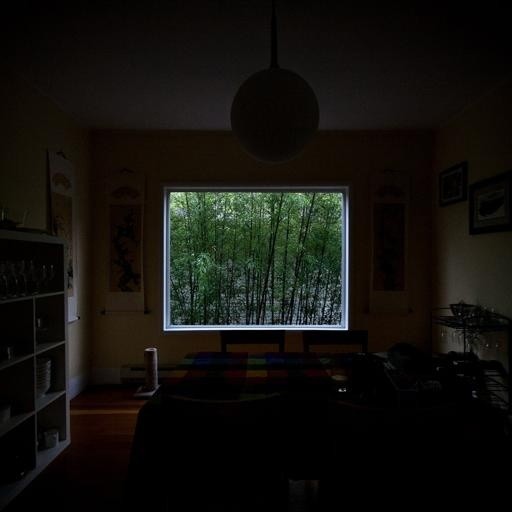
[157,387,286,512]
[220,330,286,353]
[303,330,368,353]
[324,394,498,512]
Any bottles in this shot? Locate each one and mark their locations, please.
[0,262,57,299]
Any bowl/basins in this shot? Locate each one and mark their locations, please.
[37,359,52,397]
[41,431,59,448]
[449,304,475,319]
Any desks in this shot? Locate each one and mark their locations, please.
[123,352,512,512]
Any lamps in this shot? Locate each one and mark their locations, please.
[229,0,320,166]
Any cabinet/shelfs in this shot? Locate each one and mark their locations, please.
[436,159,468,206]
[470,171,512,235]
[0,218,71,505]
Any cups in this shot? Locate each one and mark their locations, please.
[0,405,11,428]
[4,344,16,361]
